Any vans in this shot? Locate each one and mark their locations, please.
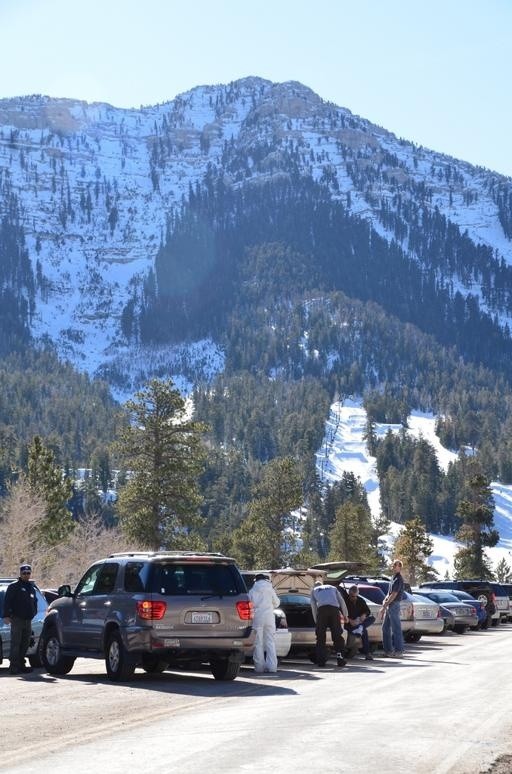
[0,577,51,667]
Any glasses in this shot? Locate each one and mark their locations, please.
[22,572,30,576]
[350,595,358,598]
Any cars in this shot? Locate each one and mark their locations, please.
[239,561,511,665]
[40,588,61,605]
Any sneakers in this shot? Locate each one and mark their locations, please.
[382,651,404,658]
[351,629,362,637]
[366,654,373,660]
[313,659,326,666]
[337,658,346,667]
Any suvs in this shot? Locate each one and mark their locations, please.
[39,550,257,684]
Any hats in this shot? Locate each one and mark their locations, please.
[19,565,31,574]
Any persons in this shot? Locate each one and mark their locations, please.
[248,574,281,673]
[344,586,374,661]
[484,591,497,628]
[310,581,349,667]
[378,559,405,659]
[3,564,38,676]
